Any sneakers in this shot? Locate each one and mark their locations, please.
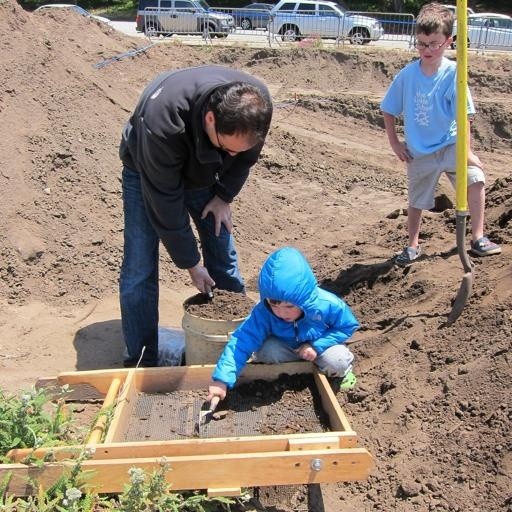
[396,244,421,266]
[471,236,501,256]
[332,373,356,392]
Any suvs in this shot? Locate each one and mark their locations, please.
[267,0,384,47]
[135,0,236,40]
[442,4,474,18]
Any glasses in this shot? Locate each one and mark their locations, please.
[216,130,234,153]
[414,37,447,52]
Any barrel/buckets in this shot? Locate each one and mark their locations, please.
[182,298,249,364]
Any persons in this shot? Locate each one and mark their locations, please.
[379,1,502,267]
[206,246,360,402]
[119,65,273,368]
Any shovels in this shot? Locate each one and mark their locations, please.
[199,396,220,434]
[446,0,475,328]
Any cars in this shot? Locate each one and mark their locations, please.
[33,4,113,24]
[448,13,512,50]
[232,3,274,30]
